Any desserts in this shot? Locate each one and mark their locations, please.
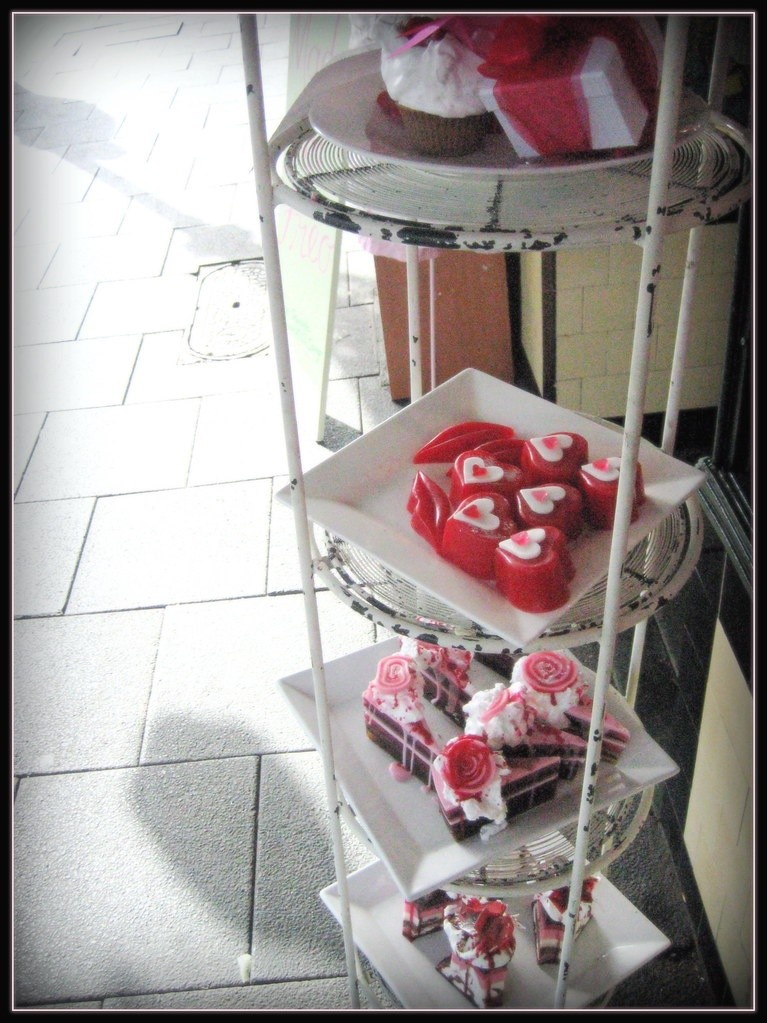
[400,875,598,1009]
[363,636,630,843]
[377,13,669,161]
[406,420,648,612]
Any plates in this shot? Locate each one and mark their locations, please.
[272,367,709,646]
[319,831,673,1010]
[277,634,682,903]
[305,70,710,175]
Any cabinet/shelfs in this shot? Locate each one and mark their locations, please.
[240,13,754,1009]
